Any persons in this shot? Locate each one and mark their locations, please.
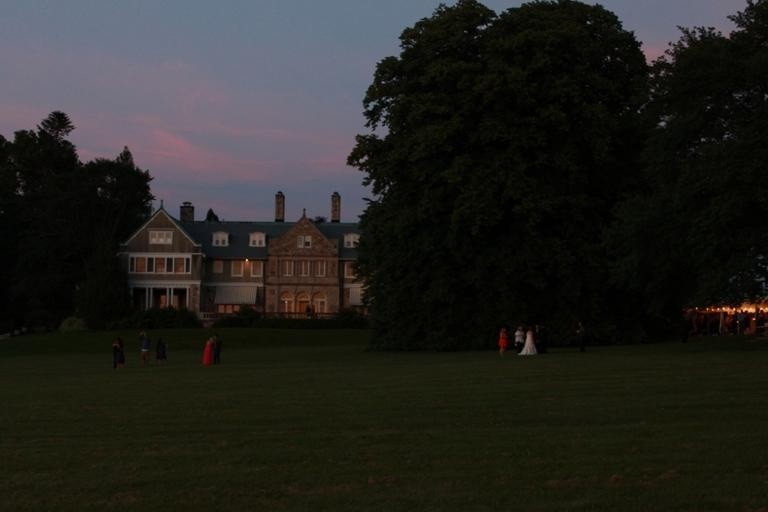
[515,326,525,349]
[576,321,586,352]
[138,330,151,368]
[534,325,545,353]
[214,334,223,365]
[517,326,538,356]
[726,308,766,336]
[202,336,214,366]
[498,327,509,353]
[112,337,126,369]
[305,304,312,320]
[155,338,167,365]
